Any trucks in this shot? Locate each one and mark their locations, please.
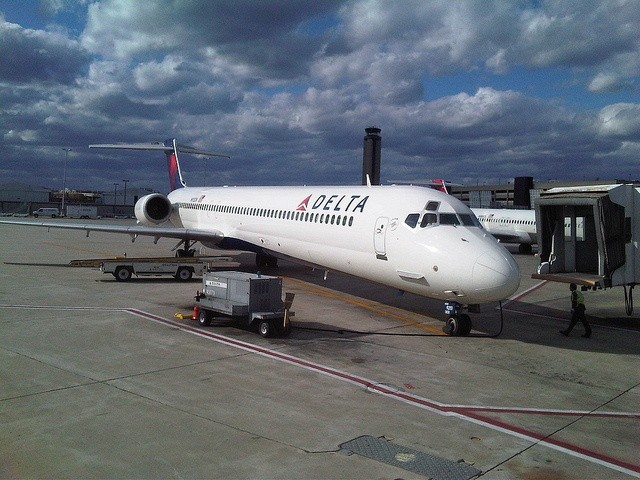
[59,205,97,219]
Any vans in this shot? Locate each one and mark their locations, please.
[33,208,59,218]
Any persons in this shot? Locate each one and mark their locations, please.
[560,282,592,337]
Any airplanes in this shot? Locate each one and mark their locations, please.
[387,179,640,253]
[0,137,519,336]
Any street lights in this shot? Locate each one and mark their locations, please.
[62,148,71,209]
[113,183,119,205]
[123,180,129,205]
